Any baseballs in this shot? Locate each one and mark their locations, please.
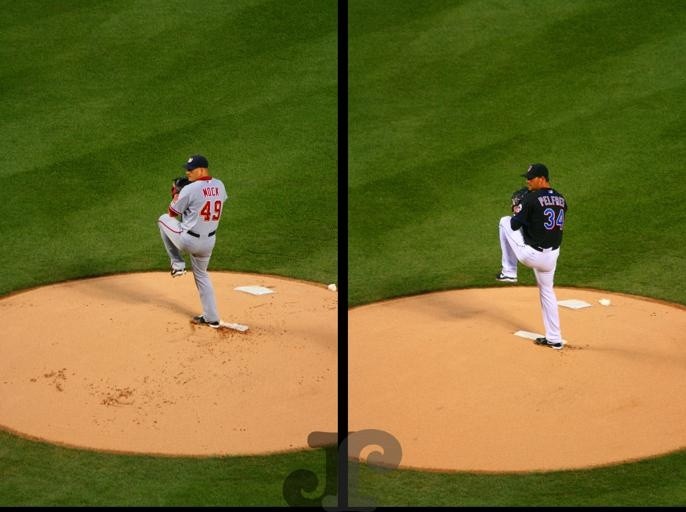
[327,284,337,292]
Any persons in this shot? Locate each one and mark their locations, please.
[157,156,227,328]
[496,164,567,349]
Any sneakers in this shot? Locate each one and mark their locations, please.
[495,273,518,283]
[171,267,186,278]
[535,337,563,349]
[193,314,220,328]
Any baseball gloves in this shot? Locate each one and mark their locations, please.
[512,187,531,212]
[172,177,192,194]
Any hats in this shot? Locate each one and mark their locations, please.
[182,155,208,170]
[520,164,549,179]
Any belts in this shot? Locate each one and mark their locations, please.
[187,230,216,238]
[530,244,560,252]
